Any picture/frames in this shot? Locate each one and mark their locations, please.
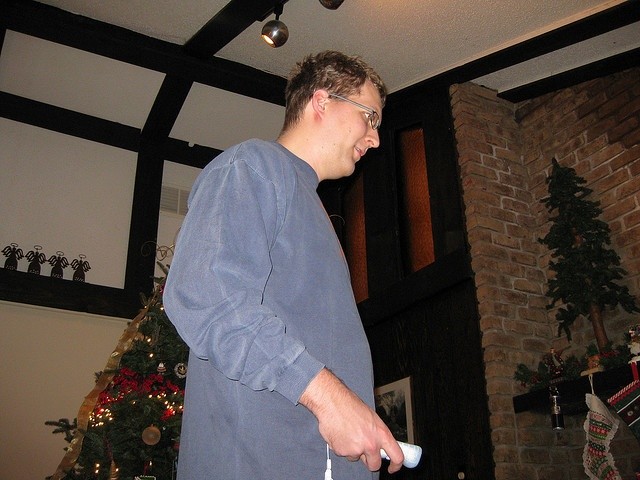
[374,374,417,444]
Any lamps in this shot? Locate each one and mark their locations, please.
[261,0,289,49]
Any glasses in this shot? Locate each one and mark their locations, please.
[329,92,381,131]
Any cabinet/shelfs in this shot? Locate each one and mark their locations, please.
[0,267,125,319]
[513,361,640,414]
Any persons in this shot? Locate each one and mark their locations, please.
[162,49,405,480]
[374,390,398,418]
